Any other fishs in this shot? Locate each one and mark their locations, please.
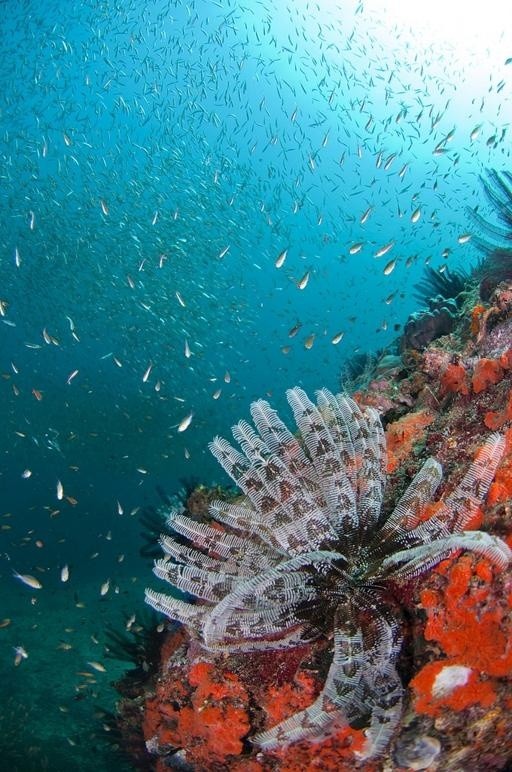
[0,0,510,772]
